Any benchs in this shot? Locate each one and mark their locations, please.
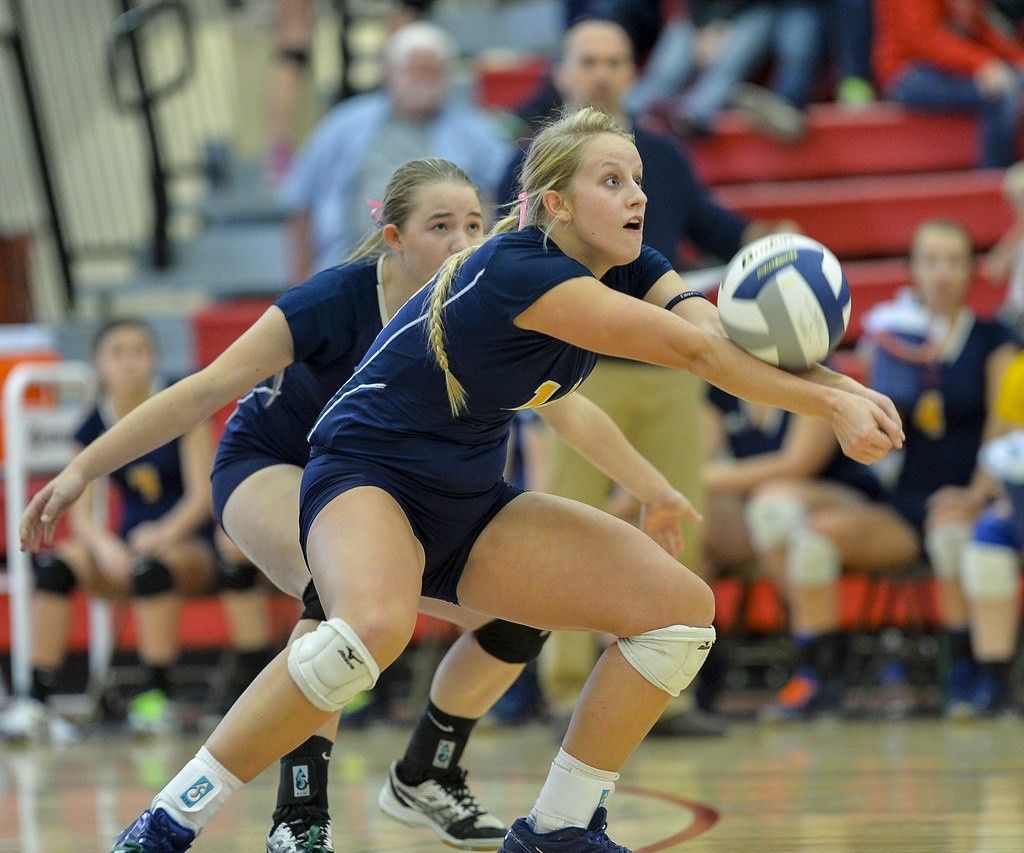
[0,104,1024,654]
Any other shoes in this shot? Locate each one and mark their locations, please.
[969,147,1022,179]
[942,664,1013,717]
[669,109,709,140]
[128,687,183,735]
[649,704,728,740]
[1,693,51,739]
[761,650,850,728]
[831,73,877,107]
[734,81,807,142]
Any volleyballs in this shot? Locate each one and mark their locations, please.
[714,230,852,371]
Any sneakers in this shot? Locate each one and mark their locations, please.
[262,814,336,852]
[497,809,632,853]
[109,796,202,853]
[379,754,509,849]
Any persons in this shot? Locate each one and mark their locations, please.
[112,106,907,851]
[20,160,708,853]
[56,0,1024,727]
